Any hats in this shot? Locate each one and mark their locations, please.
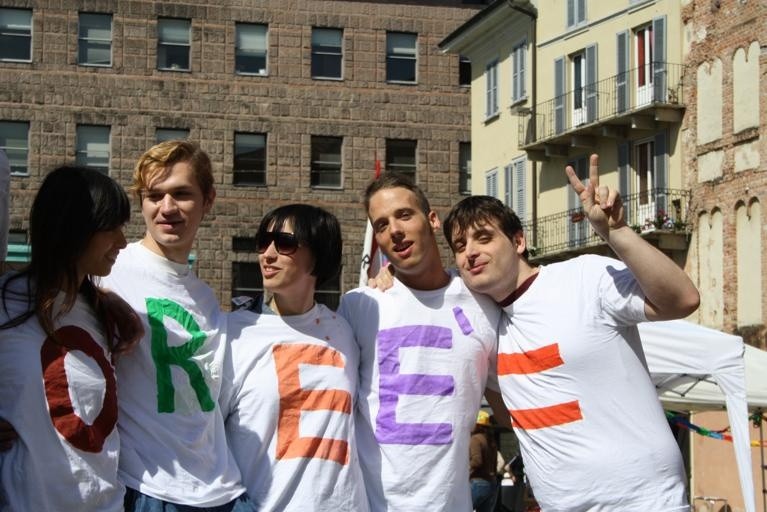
[476,410,492,426]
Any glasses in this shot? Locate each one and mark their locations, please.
[254,232,302,255]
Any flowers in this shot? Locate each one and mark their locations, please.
[630,207,671,234]
